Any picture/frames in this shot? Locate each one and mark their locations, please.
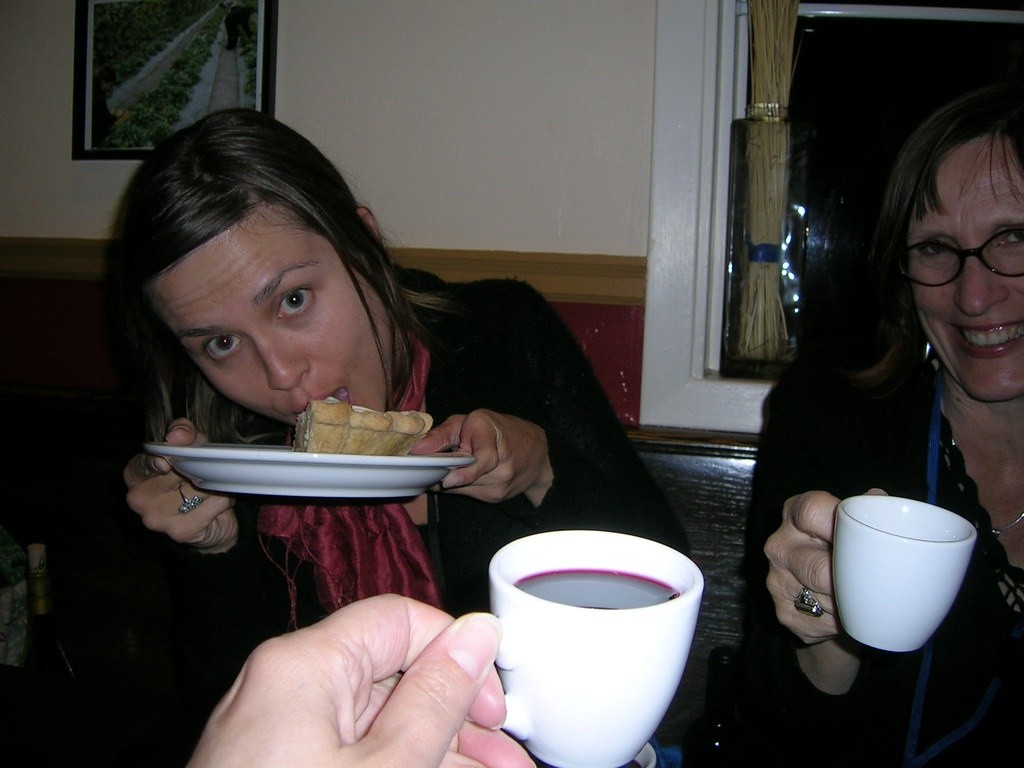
[71,0,279,162]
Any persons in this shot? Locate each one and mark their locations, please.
[740,85,1024,768]
[91,105,693,768]
[185,593,538,768]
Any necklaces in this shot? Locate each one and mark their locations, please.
[992,511,1024,535]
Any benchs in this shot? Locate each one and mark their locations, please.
[0,381,760,743]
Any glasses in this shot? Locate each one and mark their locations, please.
[898,227,1023,285]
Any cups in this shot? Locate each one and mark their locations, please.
[832,495,977,652]
[466,530,703,768]
[618,742,656,768]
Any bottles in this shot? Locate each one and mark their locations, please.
[24,543,74,698]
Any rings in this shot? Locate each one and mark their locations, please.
[176,484,201,515]
[794,587,824,617]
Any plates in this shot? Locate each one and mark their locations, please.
[144,442,476,496]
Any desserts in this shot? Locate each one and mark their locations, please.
[289,396,433,457]
